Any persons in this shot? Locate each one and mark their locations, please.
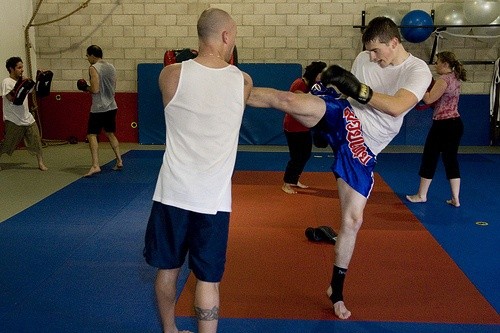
[76,45,124,179]
[242,18,432,321]
[0,57,50,173]
[406,51,468,209]
[281,61,328,195]
[142,7,253,332]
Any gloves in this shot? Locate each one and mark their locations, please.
[305,225,338,245]
[77,79,89,93]
[321,65,373,104]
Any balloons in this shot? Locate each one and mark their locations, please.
[431,2,472,38]
[368,7,401,33]
[400,10,434,44]
[462,0,500,27]
[471,16,500,43]
